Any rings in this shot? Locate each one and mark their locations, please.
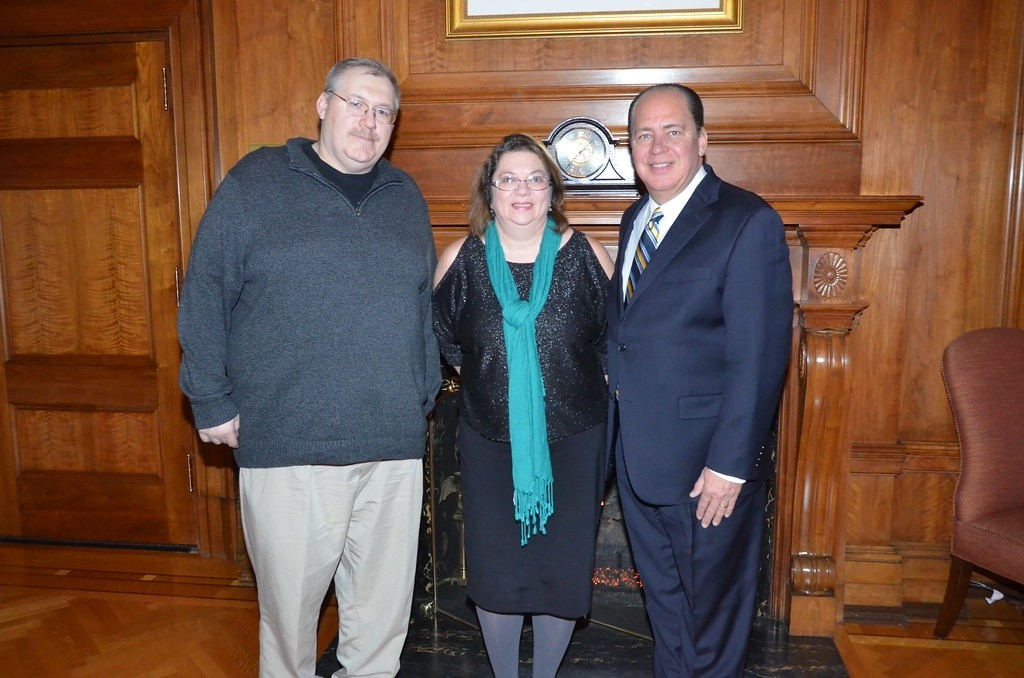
[719,504,728,508]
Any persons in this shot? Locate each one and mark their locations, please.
[177,61,437,678]
[432,134,614,678]
[608,83,794,678]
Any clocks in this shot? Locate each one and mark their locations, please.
[540,116,641,197]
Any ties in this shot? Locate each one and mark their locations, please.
[625,207,664,308]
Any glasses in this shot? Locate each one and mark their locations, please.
[327,89,399,125]
[491,175,555,191]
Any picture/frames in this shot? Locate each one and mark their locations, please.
[444,0,744,42]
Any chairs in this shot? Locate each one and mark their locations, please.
[931,325,1024,639]
